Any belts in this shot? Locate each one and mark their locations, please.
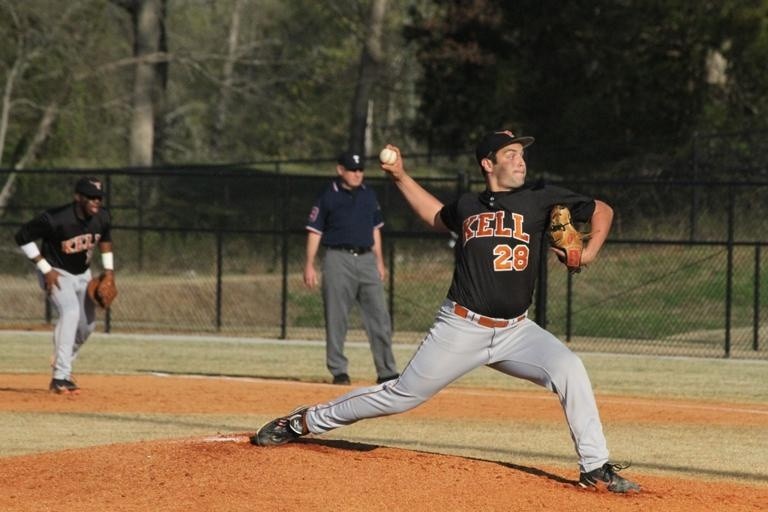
[454,304,526,328]
[328,243,373,257]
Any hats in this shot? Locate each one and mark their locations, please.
[73,177,111,199]
[475,129,534,164]
[336,150,366,171]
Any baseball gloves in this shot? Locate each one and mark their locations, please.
[547,203,587,273]
[88,273,118,309]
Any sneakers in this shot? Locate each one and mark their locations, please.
[333,373,351,386]
[577,462,642,495]
[253,403,311,447]
[50,377,77,392]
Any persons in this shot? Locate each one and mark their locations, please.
[15,177,114,395]
[304,153,401,386]
[254,131,642,494]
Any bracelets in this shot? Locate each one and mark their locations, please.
[101,252,113,269]
[36,258,52,275]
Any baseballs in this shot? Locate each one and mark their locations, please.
[378,148,397,166]
